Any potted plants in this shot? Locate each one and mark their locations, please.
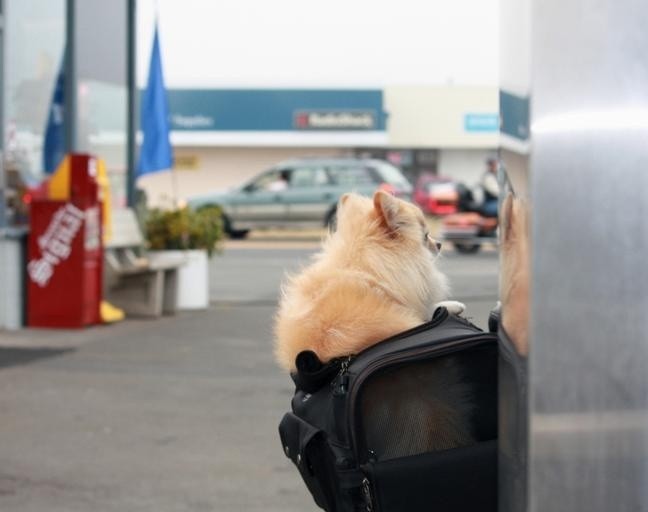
[140,201,226,311]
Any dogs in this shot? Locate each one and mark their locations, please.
[272,188,466,372]
[492,192,530,358]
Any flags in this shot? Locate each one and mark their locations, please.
[133,18,172,183]
[36,45,72,173]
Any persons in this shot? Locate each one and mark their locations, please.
[262,171,289,192]
[480,158,499,218]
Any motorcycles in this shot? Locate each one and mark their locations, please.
[423,182,496,252]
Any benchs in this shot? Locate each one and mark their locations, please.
[99,207,180,320]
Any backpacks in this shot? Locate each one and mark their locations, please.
[276,305,502,507]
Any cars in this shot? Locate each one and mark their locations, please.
[186,158,415,241]
